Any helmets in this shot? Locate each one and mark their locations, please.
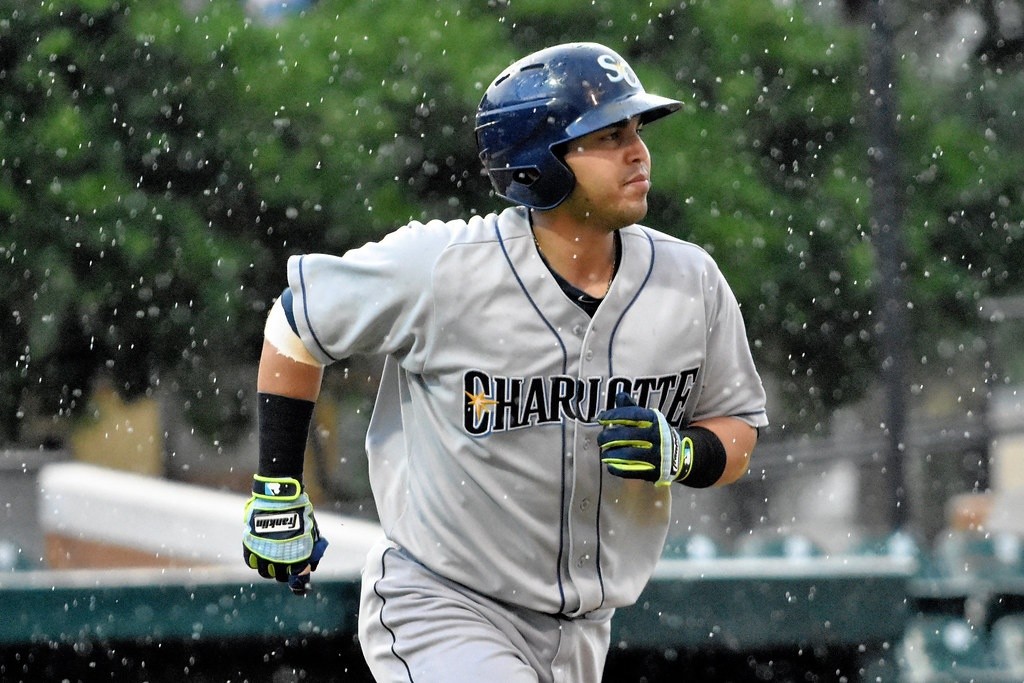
[474,42,685,211]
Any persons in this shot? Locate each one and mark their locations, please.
[243,42,771,683]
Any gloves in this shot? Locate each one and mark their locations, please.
[596,392,693,487]
[241,473,328,595]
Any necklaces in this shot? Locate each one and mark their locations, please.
[532,232,617,297]
